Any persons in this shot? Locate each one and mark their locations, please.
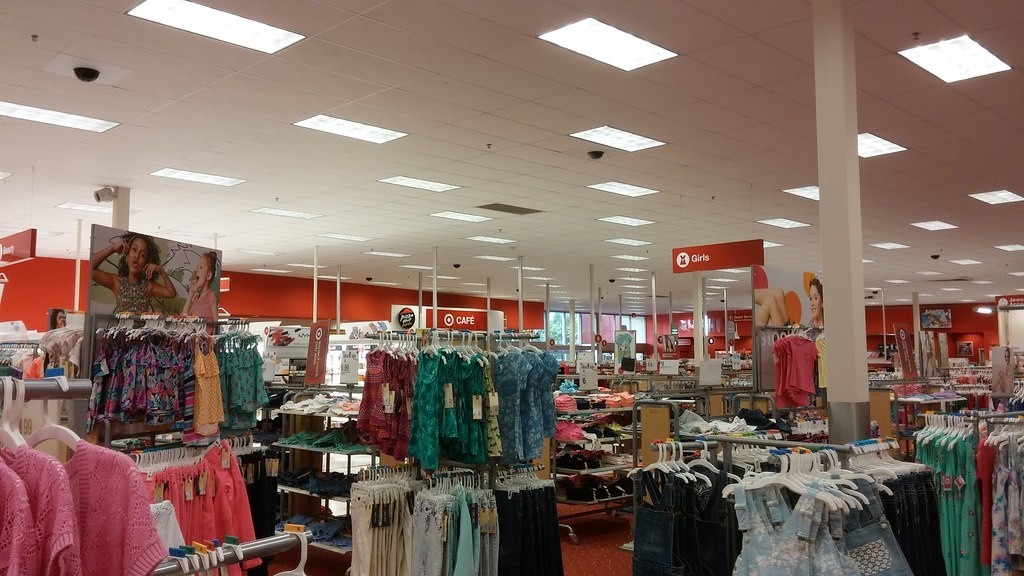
[755,278,823,326]
[50,309,66,328]
[992,348,1014,394]
[93,232,217,333]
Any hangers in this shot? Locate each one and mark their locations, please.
[163,524,309,576]
[0,308,1024,512]
